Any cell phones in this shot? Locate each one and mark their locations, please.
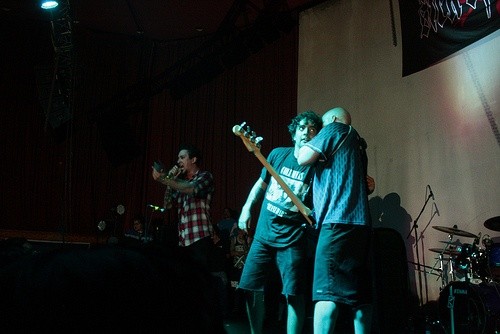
[154,162,161,174]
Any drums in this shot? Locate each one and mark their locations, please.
[436,281,500,334]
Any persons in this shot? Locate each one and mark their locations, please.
[152,144,231,327]
[294,107,374,334]
[223,207,235,218]
[126,218,144,234]
[238,111,375,334]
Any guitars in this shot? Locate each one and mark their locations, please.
[232,121,315,228]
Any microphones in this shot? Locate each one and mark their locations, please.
[166,172,174,180]
[428,185,440,216]
[148,204,166,213]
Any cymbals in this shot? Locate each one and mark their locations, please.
[484,216,500,232]
[431,226,478,239]
[429,248,463,255]
